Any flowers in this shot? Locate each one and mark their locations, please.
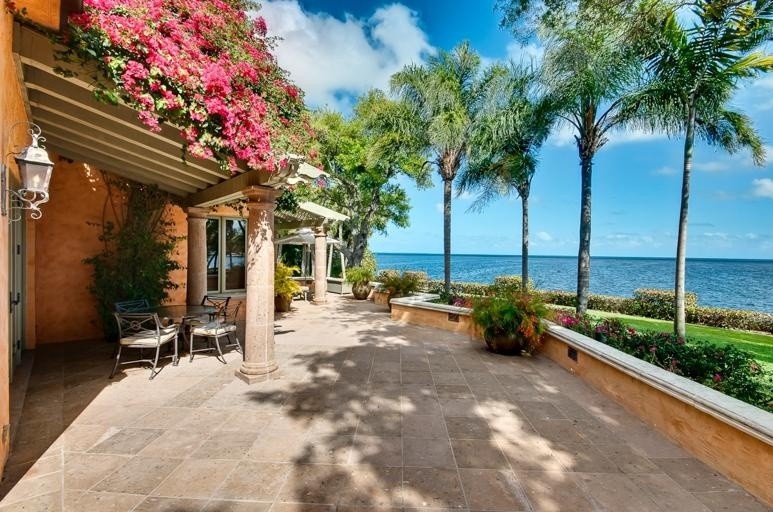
[470,275,548,353]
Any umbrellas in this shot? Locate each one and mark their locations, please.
[275,227,341,280]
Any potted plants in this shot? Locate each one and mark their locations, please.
[275,257,310,312]
[374,270,420,312]
[339,259,377,300]
[82,170,188,343]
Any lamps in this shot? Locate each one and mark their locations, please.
[0,120,54,223]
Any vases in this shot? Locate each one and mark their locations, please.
[484,323,526,355]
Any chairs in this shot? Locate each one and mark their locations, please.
[109,295,243,380]
